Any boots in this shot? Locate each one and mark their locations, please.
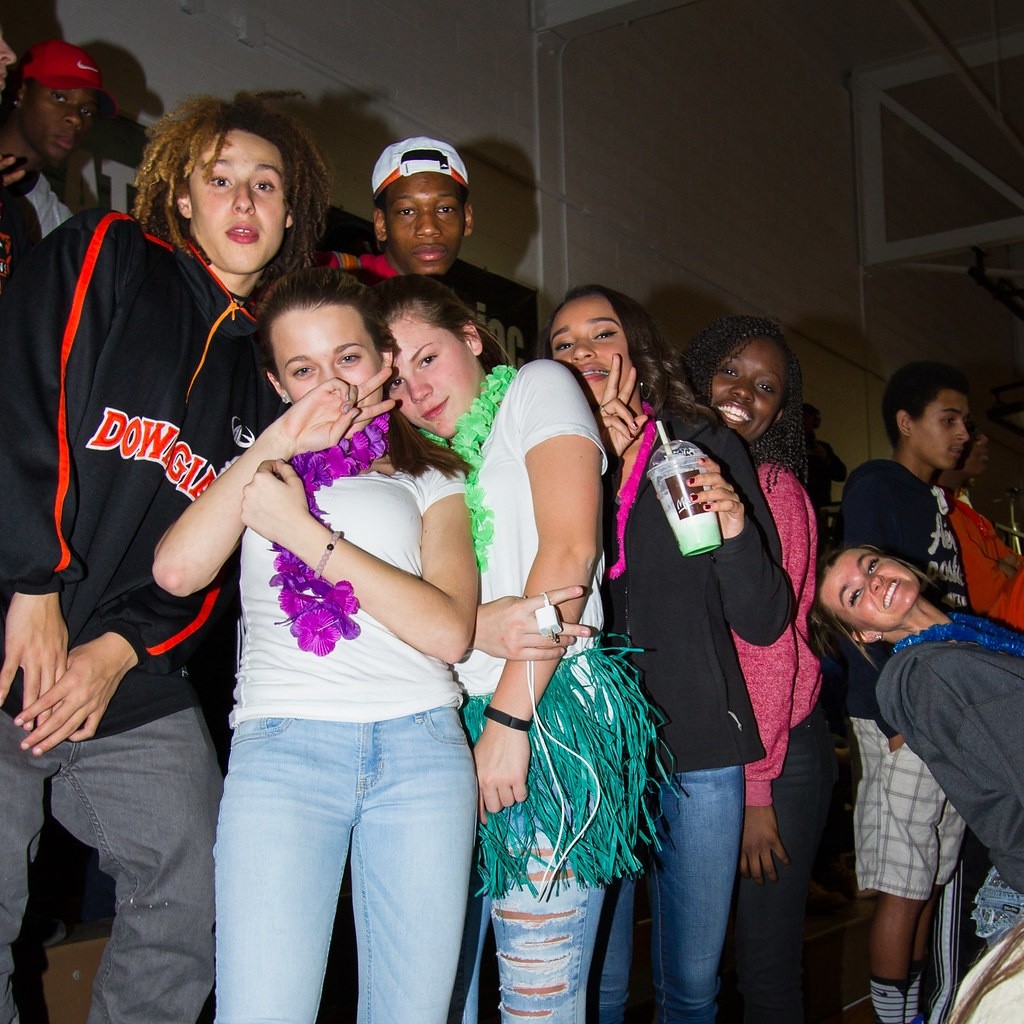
[870,960,923,1024]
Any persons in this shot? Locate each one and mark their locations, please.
[812,543,1024,894]
[542,284,823,1024]
[840,362,970,1024]
[0,91,331,1024]
[333,137,511,373]
[803,400,846,507]
[0,30,121,295]
[151,267,478,1024]
[367,273,680,1024]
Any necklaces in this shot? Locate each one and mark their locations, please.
[266,413,389,657]
[420,365,517,572]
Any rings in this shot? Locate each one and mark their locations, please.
[600,405,609,417]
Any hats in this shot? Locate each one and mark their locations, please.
[372,135,469,198]
[18,39,118,119]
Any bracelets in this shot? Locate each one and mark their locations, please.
[482,703,534,732]
[314,531,340,580]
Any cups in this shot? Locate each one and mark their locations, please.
[648,440,722,557]
